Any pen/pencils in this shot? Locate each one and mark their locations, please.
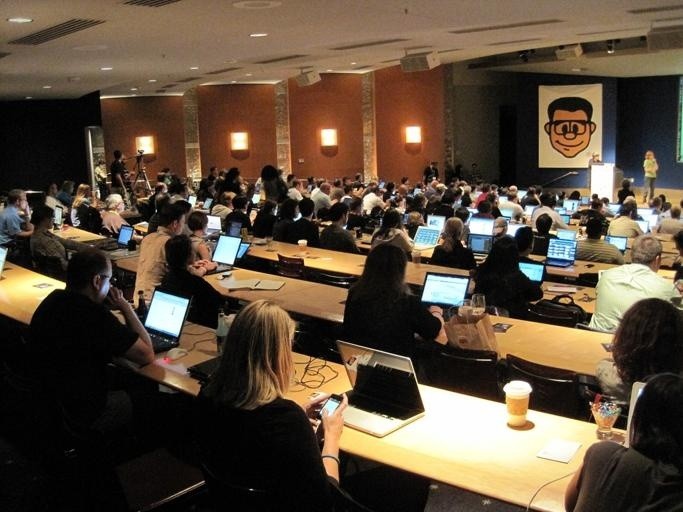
[254,281,261,287]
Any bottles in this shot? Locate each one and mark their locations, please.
[135,290,148,319]
[214,307,228,352]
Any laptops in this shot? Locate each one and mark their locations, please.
[143,285,194,353]
[54,205,63,225]
[187,193,261,275]
[0,245,9,280]
[413,188,660,285]
[418,271,472,322]
[101,224,134,250]
[320,338,428,438]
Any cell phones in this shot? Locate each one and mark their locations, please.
[316,393,344,420]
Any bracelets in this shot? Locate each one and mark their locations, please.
[318,452,340,463]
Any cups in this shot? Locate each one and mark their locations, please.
[503,379,533,428]
[470,293,486,315]
[298,239,308,255]
[591,405,621,437]
[411,251,421,268]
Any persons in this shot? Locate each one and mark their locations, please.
[119,155,135,206]
[195,299,371,511]
[0,160,683,383]
[18,247,156,453]
[109,149,131,212]
[562,370,682,512]
[639,150,659,203]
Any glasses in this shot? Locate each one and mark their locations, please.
[293,330,302,342]
[551,120,589,135]
[98,275,117,283]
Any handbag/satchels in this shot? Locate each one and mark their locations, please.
[444,305,501,358]
[529,294,586,328]
